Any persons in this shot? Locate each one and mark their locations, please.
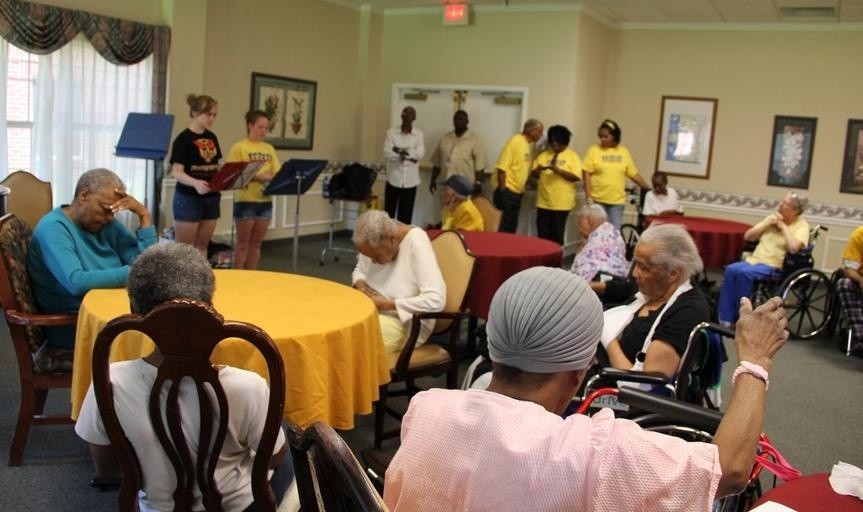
[25,168,160,489]
[347,210,448,449]
[168,95,227,258]
[225,107,282,270]
[467,224,712,417]
[719,191,810,326]
[383,106,426,225]
[382,267,790,511]
[430,110,488,231]
[74,241,304,512]
[490,119,685,291]
[833,225,862,355]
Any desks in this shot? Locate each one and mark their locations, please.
[645,216,755,268]
[70,265,391,431]
[426,231,563,356]
[751,472,863,512]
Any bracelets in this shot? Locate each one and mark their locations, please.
[729,359,770,393]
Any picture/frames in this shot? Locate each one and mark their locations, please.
[767,115,818,189]
[655,96,718,179]
[251,72,318,152]
[840,119,863,194]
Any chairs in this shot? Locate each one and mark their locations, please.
[366,231,476,449]
[1,210,78,468]
[1,171,53,230]
[285,417,393,511]
[91,298,287,509]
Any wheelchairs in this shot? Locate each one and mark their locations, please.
[568,269,764,512]
[824,263,862,359]
[619,184,653,257]
[718,223,836,341]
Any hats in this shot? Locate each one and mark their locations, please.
[484,266,607,377]
[435,175,474,197]
